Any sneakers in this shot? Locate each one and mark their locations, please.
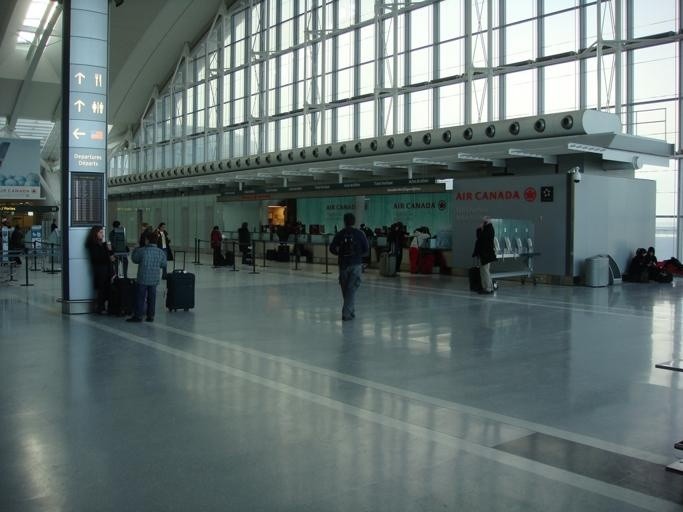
[145,317,154,322]
[126,316,143,322]
[97,310,107,314]
[342,317,352,321]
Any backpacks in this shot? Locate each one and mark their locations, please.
[340,233,358,257]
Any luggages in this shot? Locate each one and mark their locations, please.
[107,252,138,317]
[225,251,234,265]
[379,243,397,277]
[468,256,482,291]
[278,242,290,262]
[166,250,195,312]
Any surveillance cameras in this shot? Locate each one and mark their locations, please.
[573,172,581,182]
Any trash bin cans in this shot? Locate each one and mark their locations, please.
[585,255,609,287]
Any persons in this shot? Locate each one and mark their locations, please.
[154,223,173,280]
[9,224,24,249]
[50,218,57,232]
[237,222,251,264]
[210,226,225,266]
[645,246,660,281]
[84,225,115,315]
[108,221,130,279]
[125,231,167,323]
[628,247,652,273]
[329,212,370,321]
[386,222,408,272]
[471,216,497,294]
[358,222,374,237]
[138,222,152,248]
